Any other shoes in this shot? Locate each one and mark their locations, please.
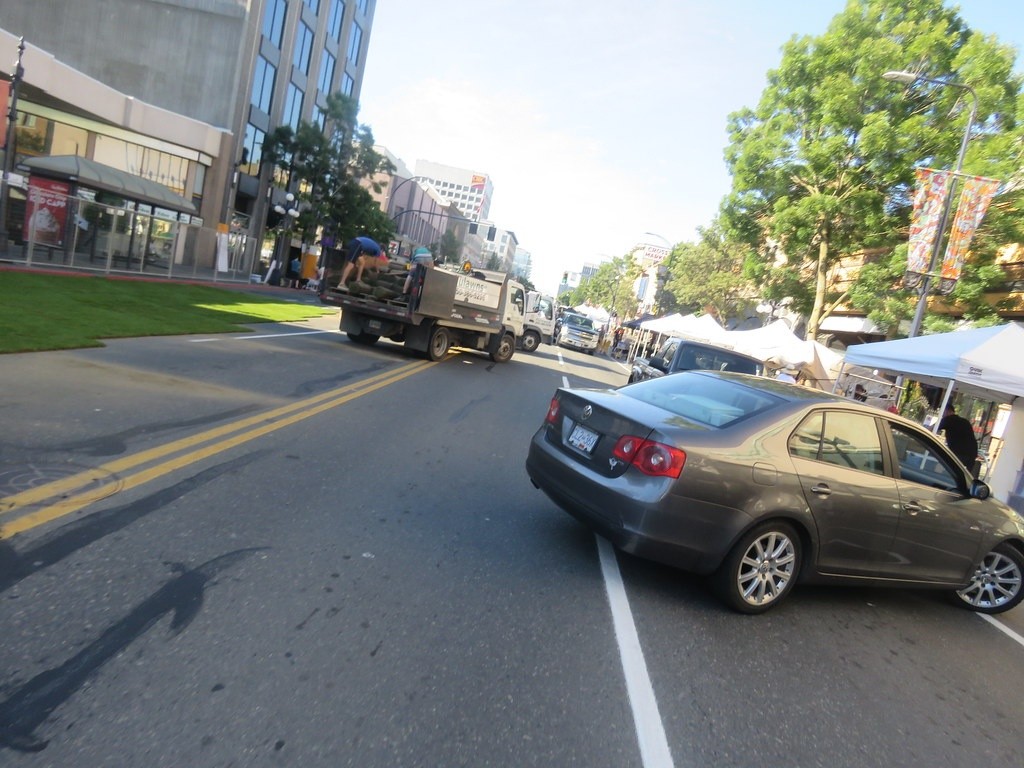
[394,295,407,302]
[337,283,349,290]
[355,280,367,287]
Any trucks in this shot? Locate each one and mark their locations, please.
[319,230,542,363]
[514,290,556,353]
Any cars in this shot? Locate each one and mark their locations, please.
[524,369,1024,617]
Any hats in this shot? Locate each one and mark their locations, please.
[781,364,799,375]
[938,403,954,410]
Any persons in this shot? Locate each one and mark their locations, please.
[611,328,621,353]
[287,257,303,289]
[392,245,434,301]
[595,325,605,346]
[337,237,388,293]
[925,403,978,474]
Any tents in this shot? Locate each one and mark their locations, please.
[573,305,894,408]
[833,318,1024,511]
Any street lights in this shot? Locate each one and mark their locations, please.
[881,69,978,414]
[268,193,301,288]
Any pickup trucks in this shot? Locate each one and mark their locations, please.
[556,306,601,356]
[626,336,766,408]
